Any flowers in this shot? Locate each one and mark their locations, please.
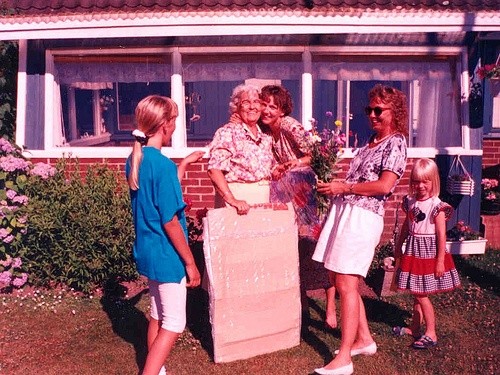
[288,110,361,222]
[476,64,500,80]
[451,220,480,242]
[98,94,114,107]
[450,172,471,182]
[481,178,498,200]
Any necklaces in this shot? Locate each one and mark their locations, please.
[374,131,397,144]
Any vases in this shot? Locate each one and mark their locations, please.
[100,105,108,112]
[389,234,488,255]
[446,158,475,197]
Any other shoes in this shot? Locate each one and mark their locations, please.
[334,341,377,356]
[159,366,166,375]
[313,361,354,375]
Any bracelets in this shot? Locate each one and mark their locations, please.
[222,190,231,199]
[350,183,355,194]
[298,159,302,167]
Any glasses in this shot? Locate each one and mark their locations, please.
[364,105,393,116]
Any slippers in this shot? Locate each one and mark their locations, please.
[393,325,422,337]
[413,336,437,348]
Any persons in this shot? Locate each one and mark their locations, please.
[228,84,337,328]
[207,86,291,216]
[125,95,207,375]
[390,158,461,349]
[311,84,409,375]
